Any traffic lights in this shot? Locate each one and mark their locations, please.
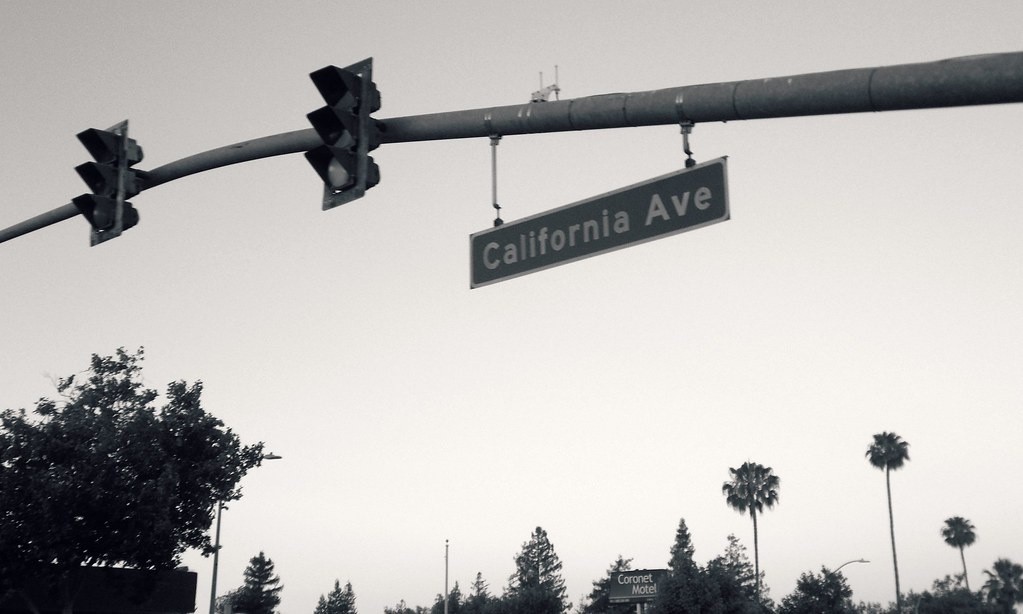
[72,120,130,244]
[302,55,374,211]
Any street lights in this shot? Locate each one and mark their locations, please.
[207,452,285,614]
